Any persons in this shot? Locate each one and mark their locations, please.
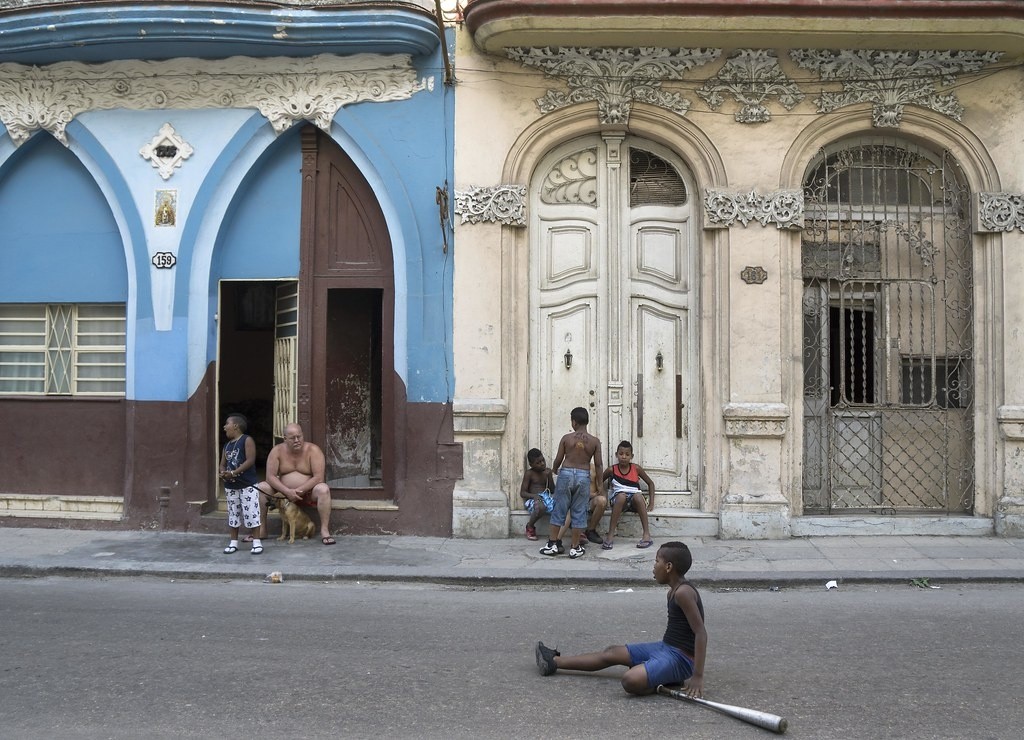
[603,440,656,550]
[555,460,609,555]
[534,540,708,700]
[240,423,336,547]
[220,413,266,555]
[520,449,557,541]
[538,406,606,559]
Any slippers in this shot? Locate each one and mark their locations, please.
[223,546,238,554]
[602,540,613,550]
[636,540,653,548]
[250,546,264,555]
[241,535,267,542]
[322,536,336,545]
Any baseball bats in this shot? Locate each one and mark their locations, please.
[655,683,787,735]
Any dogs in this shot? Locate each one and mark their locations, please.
[265,492,316,545]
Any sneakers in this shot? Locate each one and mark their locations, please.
[556,539,565,554]
[579,532,589,544]
[539,542,558,556]
[536,641,560,676]
[585,529,603,543]
[526,522,538,540]
[569,544,586,558]
